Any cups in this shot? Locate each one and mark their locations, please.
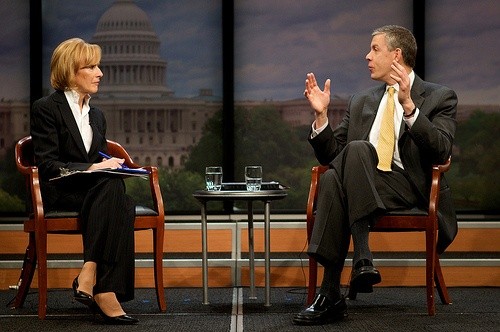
[244,166,262,192]
[205,166,223,191]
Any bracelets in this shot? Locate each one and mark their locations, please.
[403,105,416,117]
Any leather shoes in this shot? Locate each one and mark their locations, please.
[350,259,382,295]
[293,294,347,325]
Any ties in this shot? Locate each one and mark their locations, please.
[375,87,395,171]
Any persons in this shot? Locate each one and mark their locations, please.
[29,38,135,325]
[293,26,457,325]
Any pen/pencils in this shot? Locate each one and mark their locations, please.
[98,151,126,168]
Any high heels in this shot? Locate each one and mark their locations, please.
[88,297,138,325]
[71,277,93,305]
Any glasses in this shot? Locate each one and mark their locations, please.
[76,64,101,71]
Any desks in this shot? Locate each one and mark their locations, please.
[192,190,288,308]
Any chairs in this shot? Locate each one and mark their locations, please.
[302,153,453,316]
[6,135,167,320]
